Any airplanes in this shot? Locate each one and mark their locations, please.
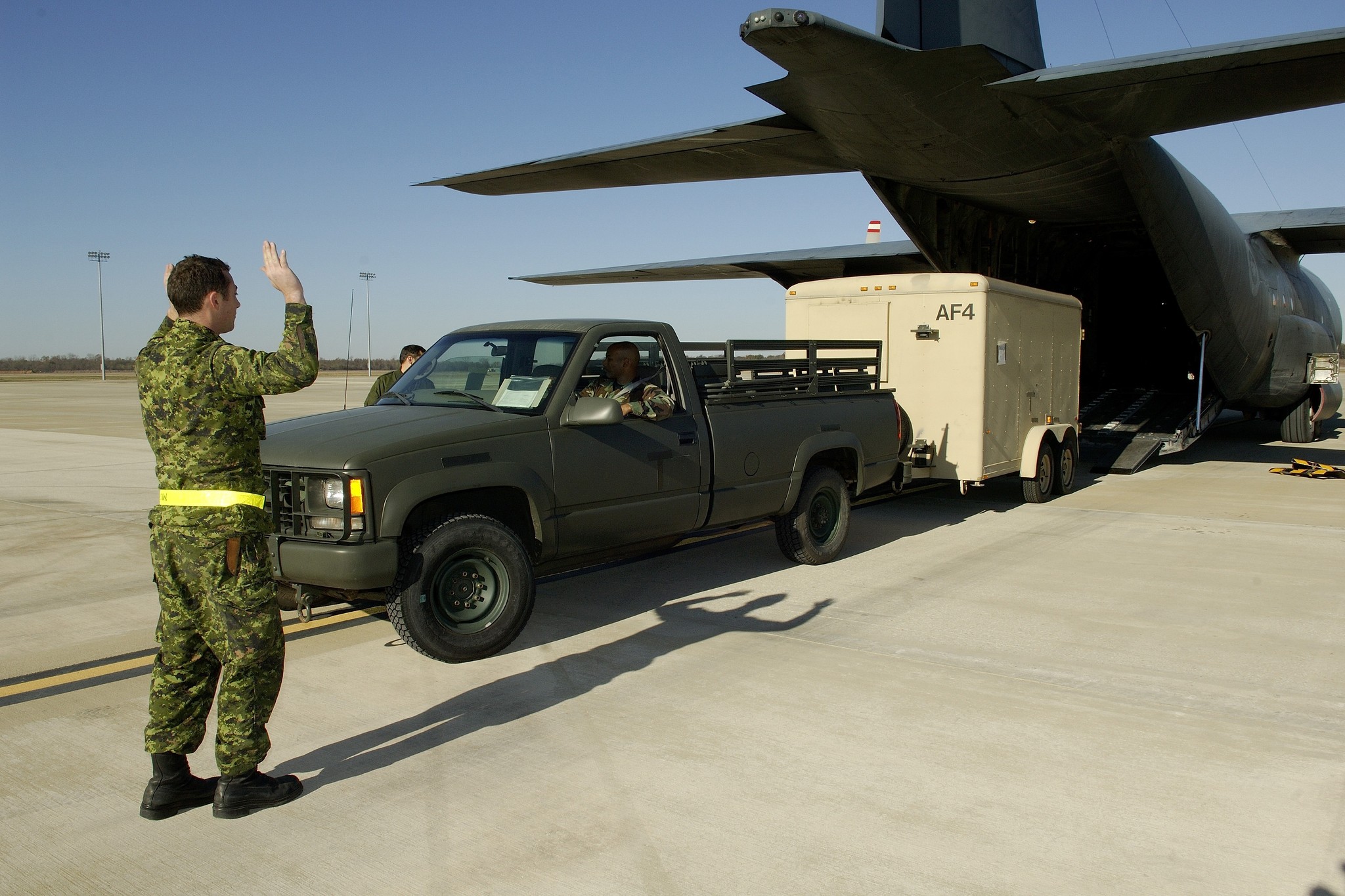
[404,0,1344,475]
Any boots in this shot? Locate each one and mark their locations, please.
[140,752,222,821]
[211,760,304,819]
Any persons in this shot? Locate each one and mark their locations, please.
[133,240,321,822]
[572,340,675,423]
[364,345,436,408]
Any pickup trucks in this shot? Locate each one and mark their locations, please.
[258,314,911,664]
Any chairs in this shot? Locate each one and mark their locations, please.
[531,365,562,376]
[638,365,661,389]
